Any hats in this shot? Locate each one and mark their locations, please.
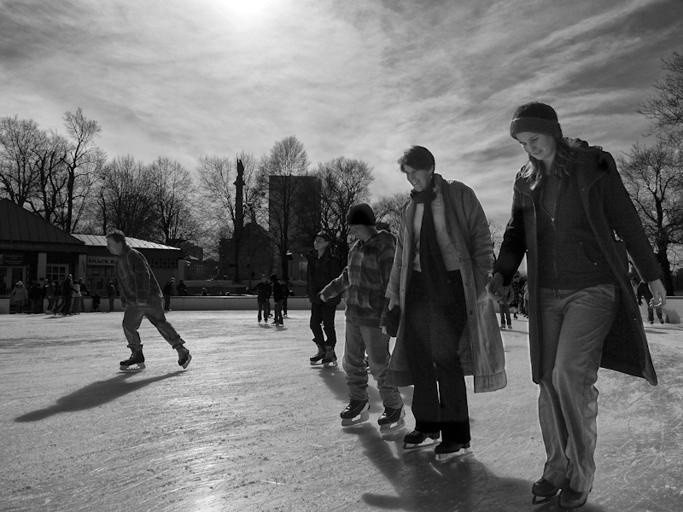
[346,203,377,226]
[510,103,563,140]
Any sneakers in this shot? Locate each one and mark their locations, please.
[341,399,368,420]
[435,440,470,454]
[378,406,401,424]
[120,351,144,365]
[532,477,558,497]
[560,482,591,509]
[178,347,189,365]
[405,430,440,443]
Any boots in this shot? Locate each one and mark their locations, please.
[310,338,337,363]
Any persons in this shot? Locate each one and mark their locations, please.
[317,203,405,425]
[306,230,341,364]
[247,272,291,325]
[496,268,664,330]
[105,230,189,366]
[10,272,190,317]
[488,102,667,510]
[384,145,508,455]
